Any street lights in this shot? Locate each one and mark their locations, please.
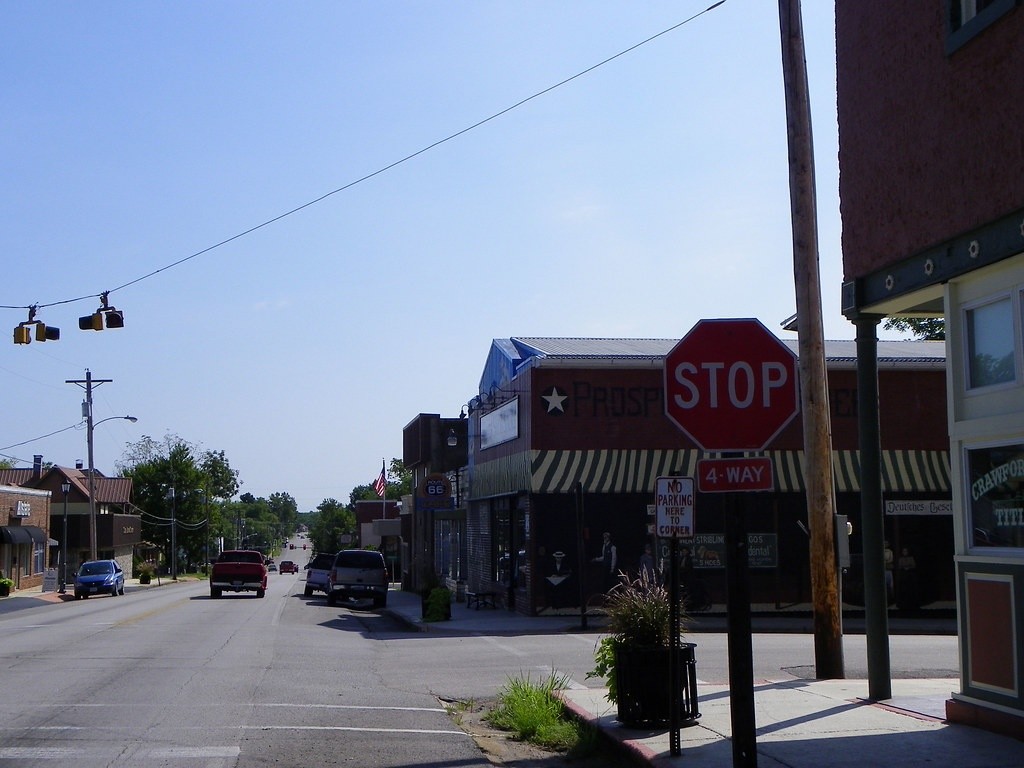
[87,415,137,561]
[57,477,71,593]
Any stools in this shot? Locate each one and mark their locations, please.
[466,589,497,610]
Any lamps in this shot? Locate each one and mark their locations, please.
[460,383,515,420]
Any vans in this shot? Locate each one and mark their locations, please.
[326,549,389,607]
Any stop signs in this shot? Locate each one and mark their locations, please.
[663,319,800,452]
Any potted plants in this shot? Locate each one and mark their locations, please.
[583,565,702,730]
[135,561,156,584]
[421,588,452,621]
[0,577,14,596]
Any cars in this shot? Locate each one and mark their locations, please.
[500,551,525,569]
[263,555,277,571]
[73,559,125,599]
[293,563,299,573]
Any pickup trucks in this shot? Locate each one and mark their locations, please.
[210,550,270,598]
[304,553,336,597]
[279,560,294,575]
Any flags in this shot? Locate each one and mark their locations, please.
[373,462,387,497]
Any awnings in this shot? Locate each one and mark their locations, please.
[22,525,47,543]
[0,527,31,543]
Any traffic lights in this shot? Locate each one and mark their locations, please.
[35,324,60,342]
[106,311,124,328]
[14,327,31,344]
[290,544,293,549]
[79,313,103,330]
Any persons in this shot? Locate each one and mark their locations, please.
[680,547,693,571]
[590,532,617,591]
[884,541,915,607]
[541,552,568,576]
